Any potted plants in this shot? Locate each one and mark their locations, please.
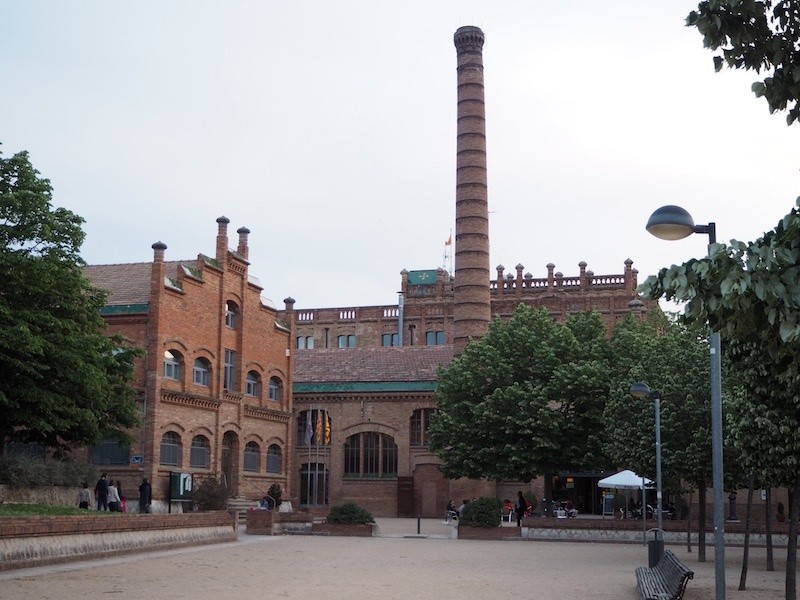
[312,501,377,536]
[454,494,522,539]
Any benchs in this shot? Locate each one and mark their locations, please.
[634,549,695,600]
[257,500,293,512]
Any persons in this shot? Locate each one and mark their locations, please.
[95,472,127,513]
[446,490,532,526]
[629,497,675,521]
[139,476,152,514]
[76,483,91,509]
[565,499,575,517]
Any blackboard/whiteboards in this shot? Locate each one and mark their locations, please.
[603,496,614,514]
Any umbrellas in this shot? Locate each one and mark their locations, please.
[598,470,655,494]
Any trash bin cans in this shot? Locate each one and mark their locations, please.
[647,540,664,567]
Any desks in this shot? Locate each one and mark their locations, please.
[553,509,568,518]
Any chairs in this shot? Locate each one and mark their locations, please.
[446,509,459,524]
[500,497,514,523]
